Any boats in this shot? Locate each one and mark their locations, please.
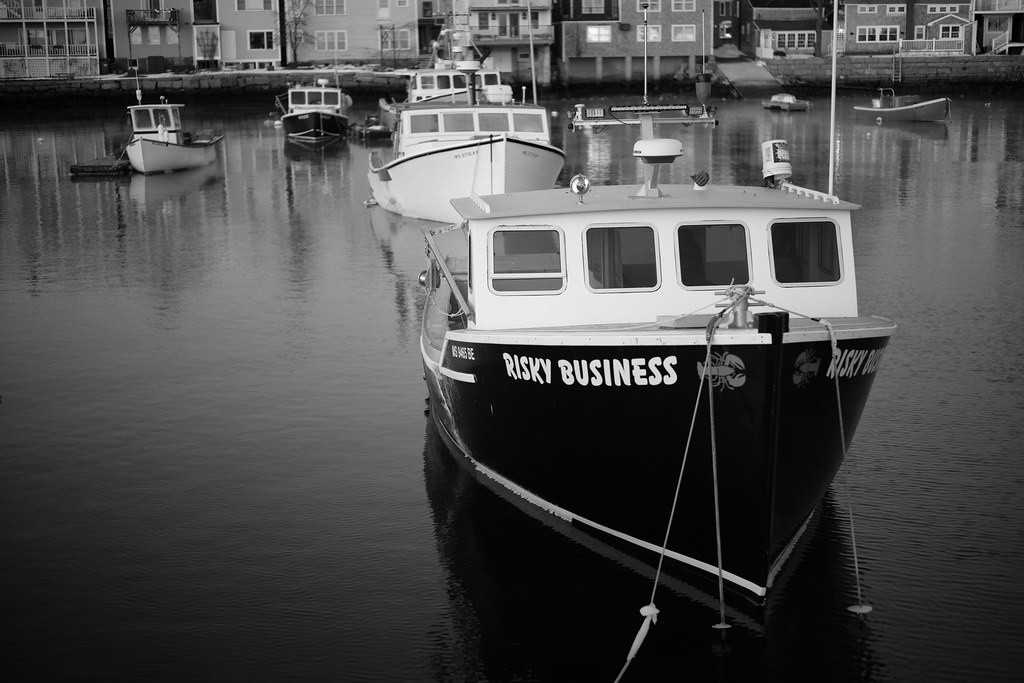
[367,1,567,224]
[762,93,814,110]
[124,57,225,177]
[419,0,898,612]
[281,78,352,152]
[852,87,953,122]
